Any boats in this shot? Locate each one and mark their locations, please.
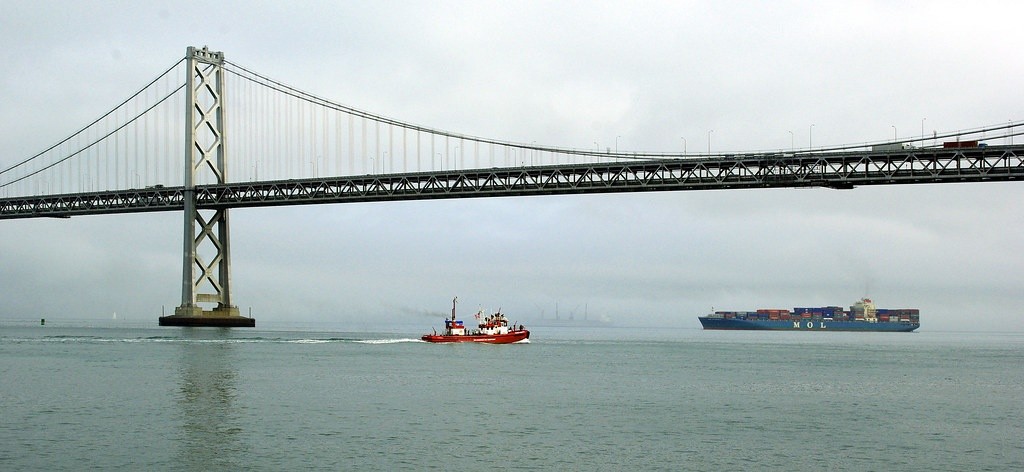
[421,294,530,346]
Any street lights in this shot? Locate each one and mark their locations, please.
[594,142,599,163]
[455,147,459,170]
[708,130,713,158]
[681,137,686,158]
[46,182,49,194]
[135,173,140,189]
[512,149,516,167]
[530,141,536,166]
[892,126,897,141]
[383,151,387,174]
[810,124,814,153]
[309,162,314,177]
[317,156,322,178]
[615,136,621,162]
[252,160,261,181]
[790,131,794,153]
[371,158,375,176]
[922,118,926,149]
[437,153,442,171]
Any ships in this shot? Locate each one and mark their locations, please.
[697,296,920,332]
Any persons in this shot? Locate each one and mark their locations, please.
[513,321,517,331]
[464,328,467,336]
[432,327,436,336]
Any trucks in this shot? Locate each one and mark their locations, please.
[943,142,989,148]
[145,185,163,189]
[873,144,913,152]
